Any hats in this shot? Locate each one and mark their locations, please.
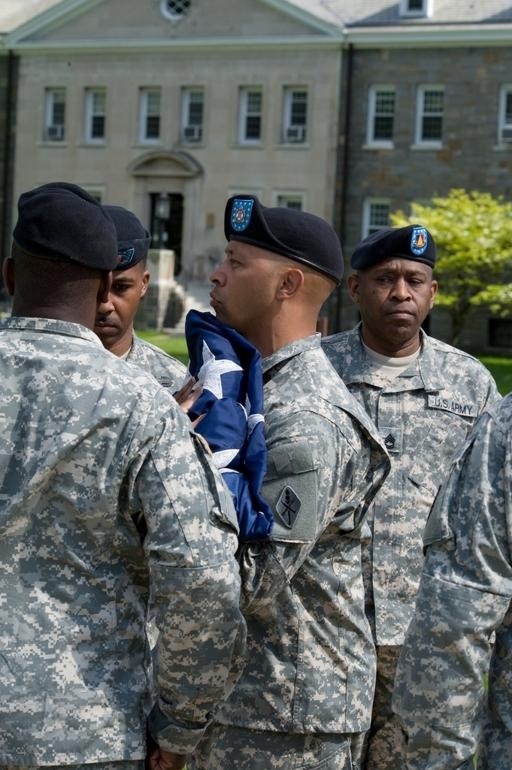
[101,204,153,271]
[351,224,436,271]
[224,195,345,289]
[12,182,119,273]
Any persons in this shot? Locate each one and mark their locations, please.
[166,192,393,770]
[1,180,252,769]
[319,222,502,770]
[366,389,511,770]
[97,206,193,392]
